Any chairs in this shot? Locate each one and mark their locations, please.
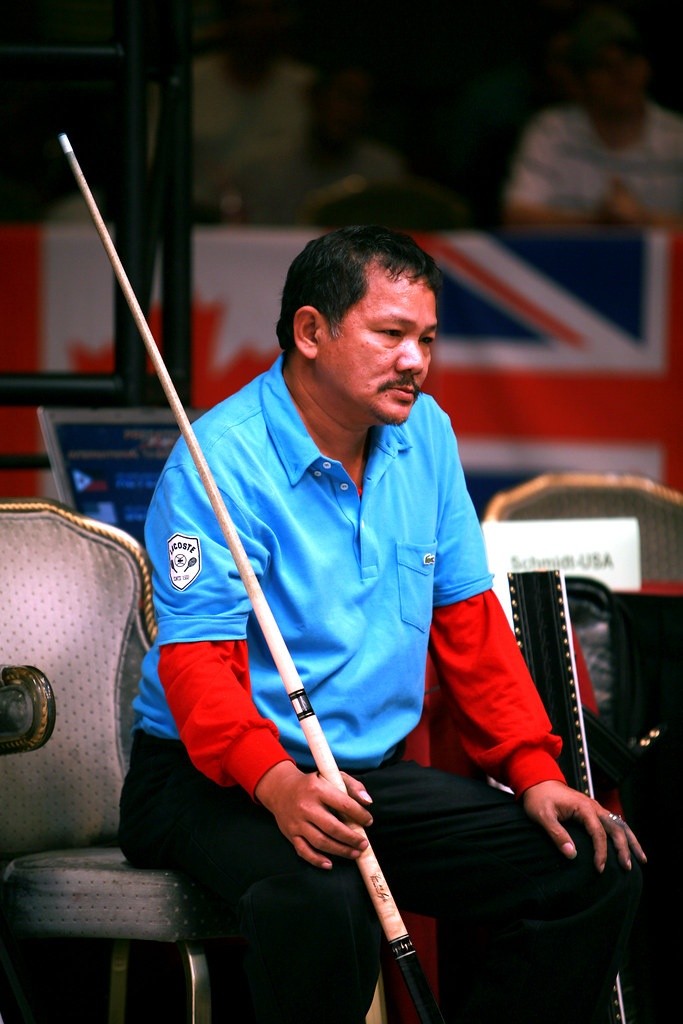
[486,471,683,581]
[0,494,386,1024]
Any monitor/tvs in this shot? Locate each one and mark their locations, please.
[36,407,208,554]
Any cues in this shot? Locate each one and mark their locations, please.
[58,134,445,1024]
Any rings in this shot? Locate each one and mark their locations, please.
[609,812,619,823]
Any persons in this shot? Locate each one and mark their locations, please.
[116,228,650,1024]
